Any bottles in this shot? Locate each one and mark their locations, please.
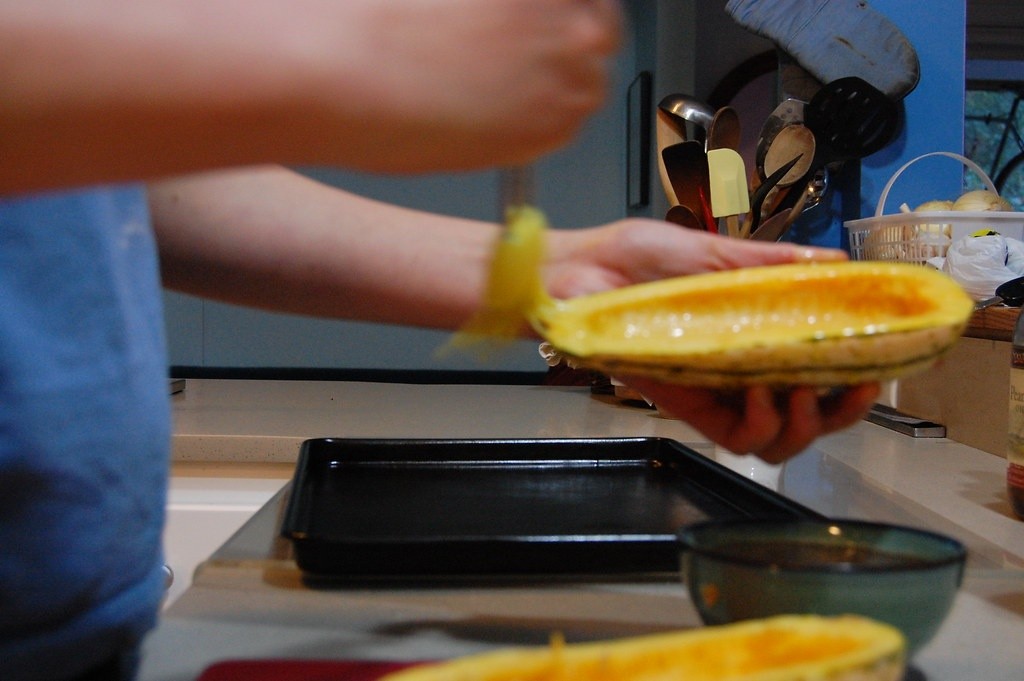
[1007,305,1024,523]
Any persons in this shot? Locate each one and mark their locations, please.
[0,1,880,681]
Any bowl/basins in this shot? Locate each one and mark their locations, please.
[676,519,967,661]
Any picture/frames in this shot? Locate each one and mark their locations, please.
[625,70,649,211]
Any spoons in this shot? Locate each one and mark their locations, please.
[660,104,816,240]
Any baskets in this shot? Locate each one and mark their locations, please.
[841,150,1024,278]
